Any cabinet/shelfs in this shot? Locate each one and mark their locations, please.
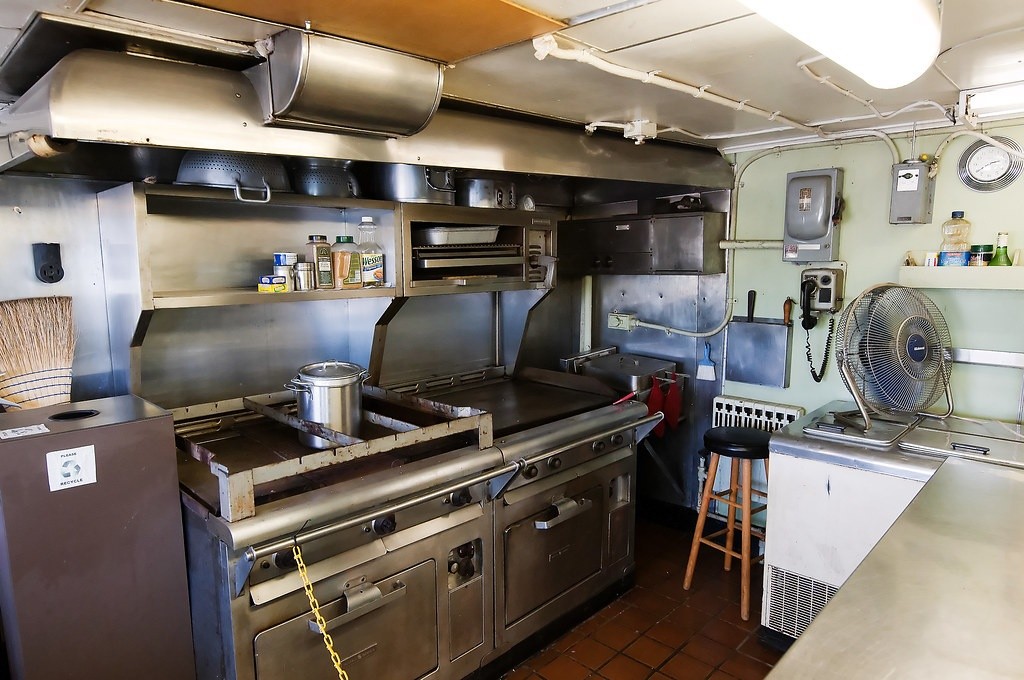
[554,211,727,275]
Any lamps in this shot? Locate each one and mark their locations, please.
[736,0,945,93]
[959,79,1024,127]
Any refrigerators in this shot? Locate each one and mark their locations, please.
[760,399,1024,640]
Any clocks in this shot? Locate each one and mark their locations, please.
[957,134,1024,193]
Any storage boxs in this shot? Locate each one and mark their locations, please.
[421,226,501,244]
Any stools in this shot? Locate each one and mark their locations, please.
[679,422,772,623]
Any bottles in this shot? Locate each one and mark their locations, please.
[303,234,334,289]
[330,235,363,290]
[937,210,1012,266]
[357,215,385,288]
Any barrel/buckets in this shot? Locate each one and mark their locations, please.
[285,359,371,450]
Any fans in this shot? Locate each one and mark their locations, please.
[827,280,959,434]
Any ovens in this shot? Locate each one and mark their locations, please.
[183,423,638,680]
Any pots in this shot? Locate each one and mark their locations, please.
[378,163,457,206]
[459,178,518,209]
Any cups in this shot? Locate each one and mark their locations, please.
[274,265,295,291]
[293,262,315,290]
